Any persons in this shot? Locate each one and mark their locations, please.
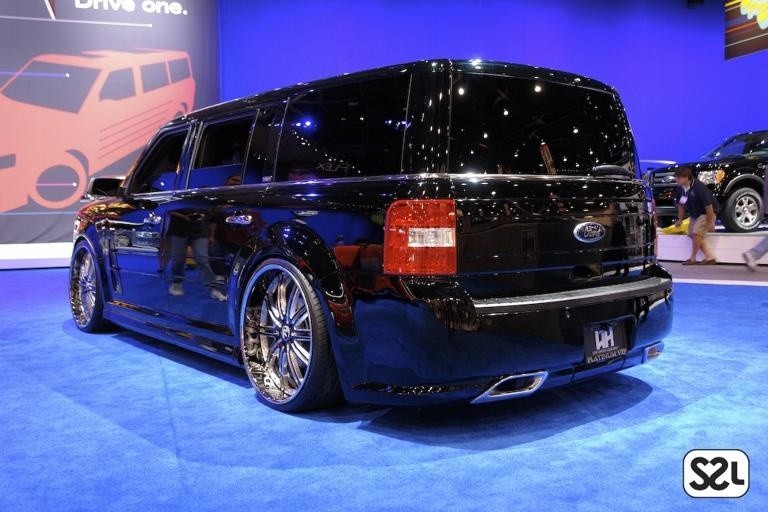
[155,174,244,301]
[674,167,720,266]
[741,233,768,272]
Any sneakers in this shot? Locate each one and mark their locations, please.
[742,249,757,272]
[697,258,717,265]
[209,288,226,302]
[169,287,184,296]
[682,259,696,264]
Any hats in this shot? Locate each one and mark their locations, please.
[673,167,691,176]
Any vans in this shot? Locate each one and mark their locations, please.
[69,58,676,414]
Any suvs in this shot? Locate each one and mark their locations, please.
[642,131,768,234]
